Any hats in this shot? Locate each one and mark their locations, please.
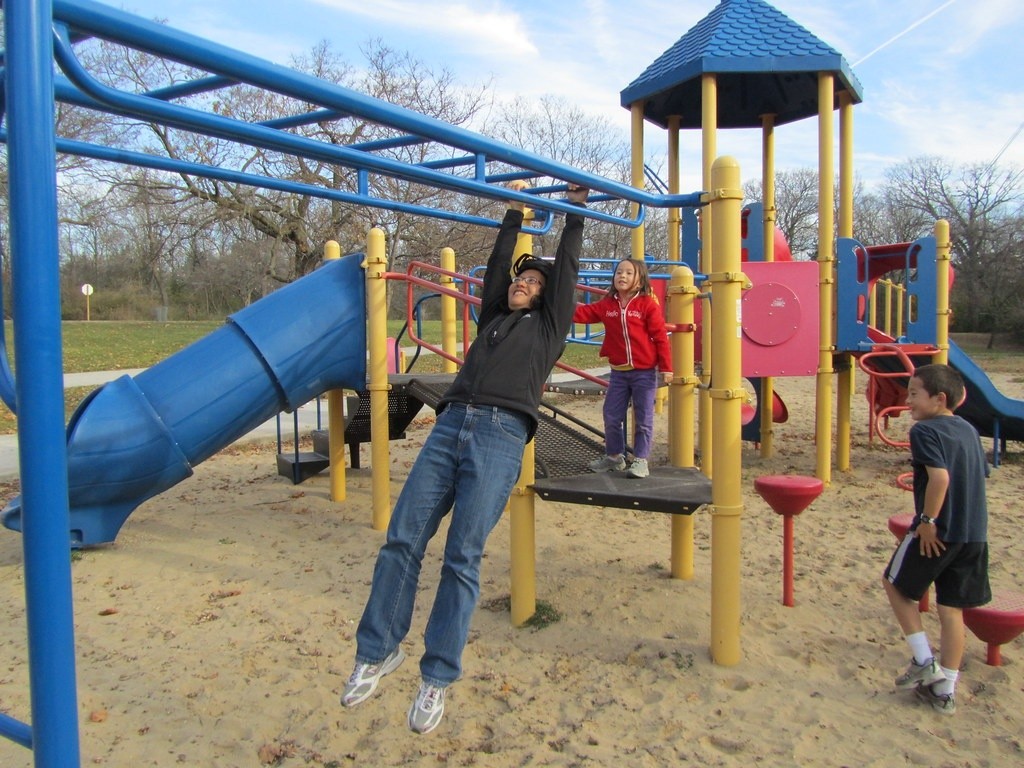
[513,253,553,276]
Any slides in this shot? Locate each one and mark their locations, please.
[1,248,365,549]
[741,208,789,424]
[872,333,1024,442]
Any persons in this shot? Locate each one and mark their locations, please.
[338,178,589,736]
[572,258,674,478]
[879,364,993,716]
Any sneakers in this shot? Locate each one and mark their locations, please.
[408,682,448,735]
[914,685,957,716]
[338,644,406,707]
[894,656,947,689]
[587,454,626,473]
[626,459,649,477]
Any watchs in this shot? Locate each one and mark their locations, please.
[920,513,937,525]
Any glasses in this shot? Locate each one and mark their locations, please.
[512,276,544,287]
